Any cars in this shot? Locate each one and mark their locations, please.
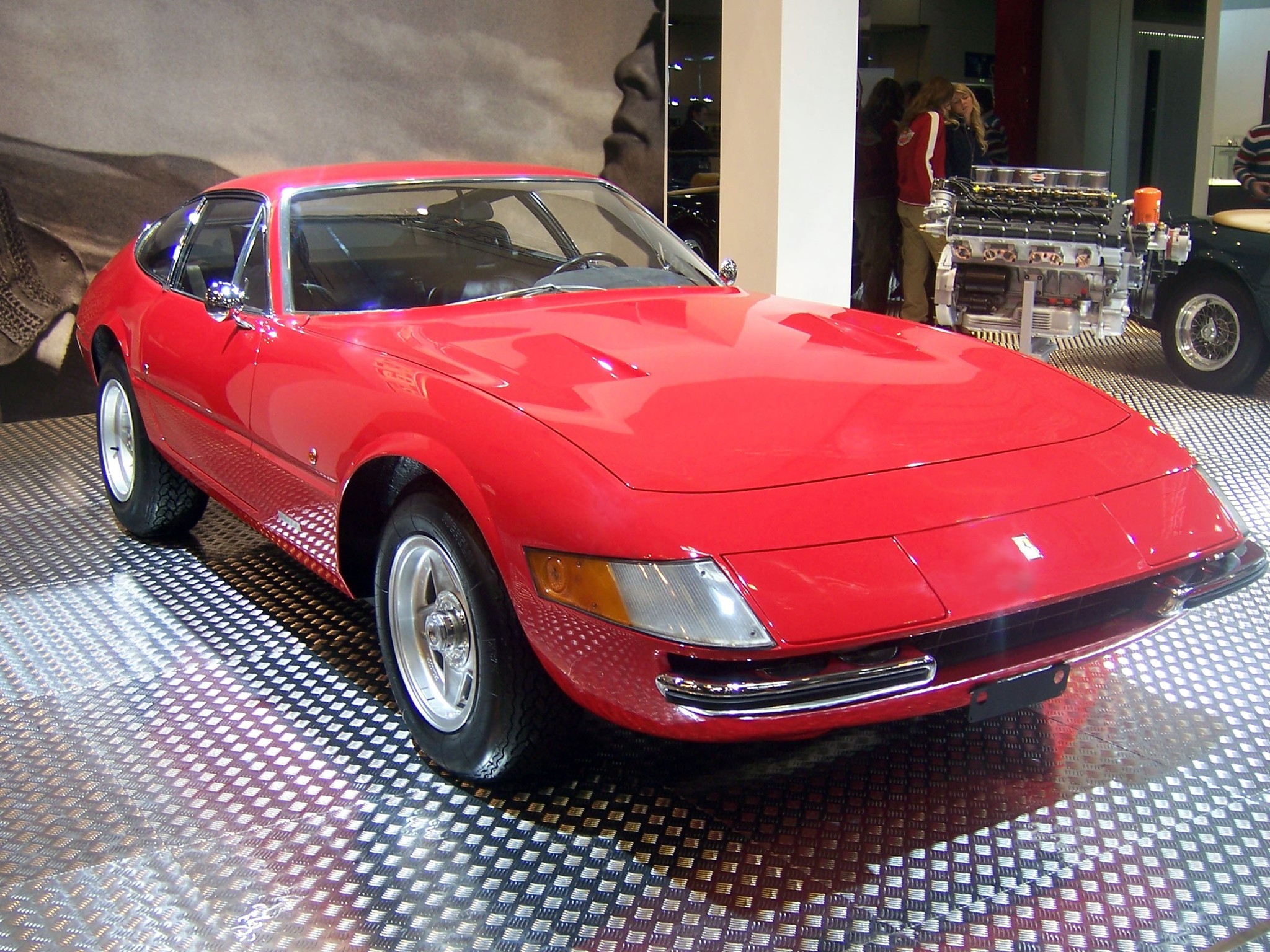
[1146,207,1269,394]
[76,160,1268,788]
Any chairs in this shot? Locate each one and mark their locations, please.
[230,224,341,311]
[424,221,530,306]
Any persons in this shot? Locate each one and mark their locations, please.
[1228,123,1270,211]
[594,0,667,268]
[854,73,988,322]
